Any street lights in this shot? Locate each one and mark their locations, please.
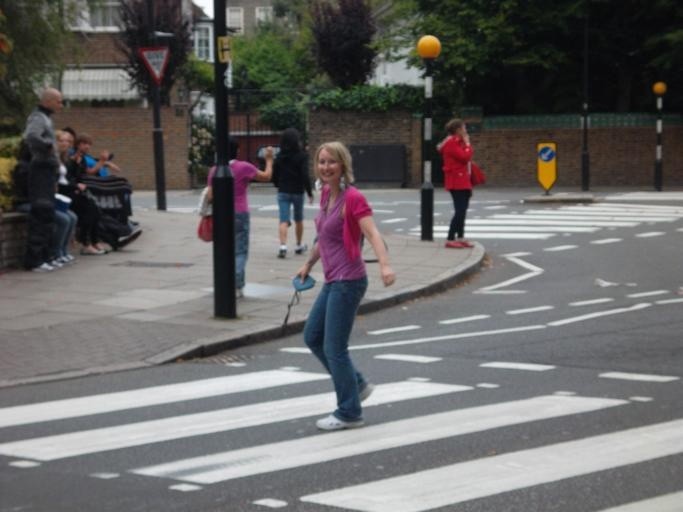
[651,81,669,191]
[414,32,442,241]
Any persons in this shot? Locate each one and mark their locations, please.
[297,141,395,430]
[272,129,313,258]
[437,119,476,249]
[208,138,273,298]
[14,87,143,273]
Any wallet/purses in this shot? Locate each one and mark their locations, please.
[293,274,316,292]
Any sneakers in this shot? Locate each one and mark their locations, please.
[277,245,288,259]
[294,245,308,255]
[31,252,76,274]
[444,239,476,251]
[315,382,373,430]
[236,287,245,298]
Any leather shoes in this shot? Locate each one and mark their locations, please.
[80,243,113,256]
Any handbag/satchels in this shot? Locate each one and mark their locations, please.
[197,216,214,242]
[470,163,486,186]
